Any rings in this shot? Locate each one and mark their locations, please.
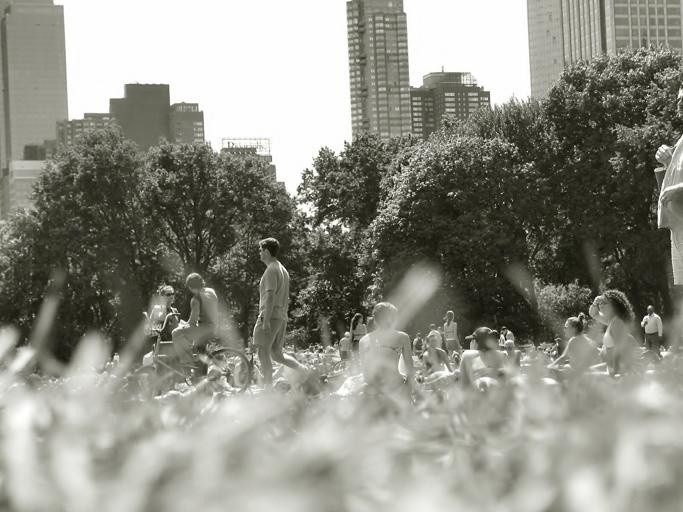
[661,197,665,201]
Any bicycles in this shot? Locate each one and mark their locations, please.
[128,310,251,397]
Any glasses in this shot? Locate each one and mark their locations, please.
[166,292,175,297]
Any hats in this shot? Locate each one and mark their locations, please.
[466,326,498,348]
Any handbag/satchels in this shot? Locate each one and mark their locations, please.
[654,167,667,191]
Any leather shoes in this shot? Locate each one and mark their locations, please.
[177,362,199,369]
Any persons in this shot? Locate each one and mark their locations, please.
[104,352,119,372]
[135,285,646,391]
[651,83,682,290]
[171,273,220,370]
[640,303,662,356]
[251,236,310,390]
